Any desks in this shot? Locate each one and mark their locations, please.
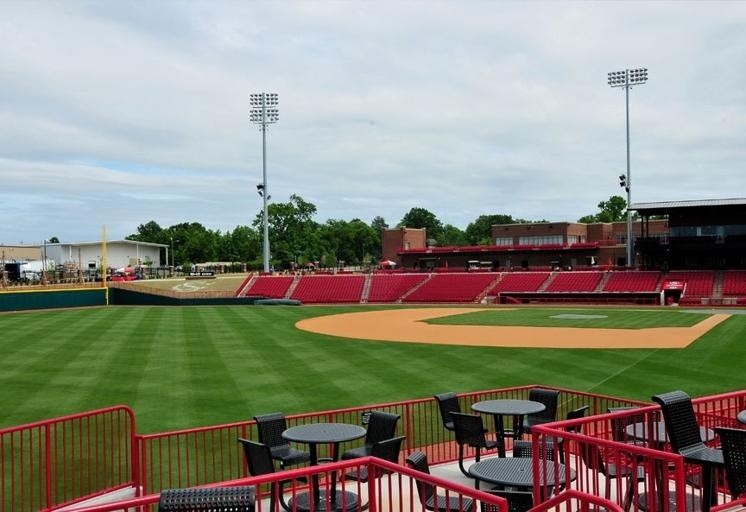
[622,420,718,511]
[282,423,368,512]
[469,400,547,458]
[737,409,746,426]
[469,458,577,511]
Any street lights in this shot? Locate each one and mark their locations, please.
[608,68,649,267]
[249,90,279,273]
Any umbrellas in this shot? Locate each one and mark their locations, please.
[378,259,398,269]
[305,263,313,270]
[114,266,135,274]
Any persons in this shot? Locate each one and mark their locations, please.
[139,268,148,280]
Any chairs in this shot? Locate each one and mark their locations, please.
[253,411,309,510]
[522,388,560,439]
[159,486,257,511]
[449,411,496,480]
[538,405,590,465]
[237,438,294,511]
[607,407,650,464]
[341,411,402,509]
[713,426,745,508]
[479,490,534,511]
[434,392,489,464]
[406,452,473,511]
[578,441,644,510]
[338,435,407,483]
[651,389,726,511]
[513,439,557,511]
